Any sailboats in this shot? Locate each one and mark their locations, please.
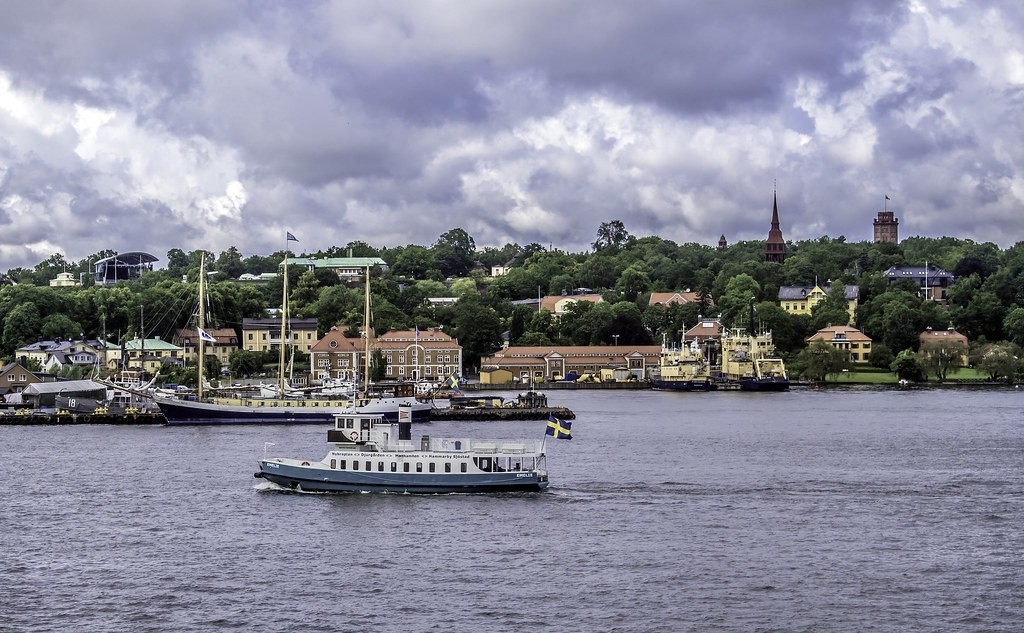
[88,250,458,425]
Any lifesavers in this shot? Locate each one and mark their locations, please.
[350,431,358,440]
[84,416,90,422]
[136,416,152,424]
[431,412,497,420]
[0,417,56,425]
[511,413,562,420]
[59,416,83,423]
[573,414,576,419]
[302,462,310,466]
[91,416,135,424]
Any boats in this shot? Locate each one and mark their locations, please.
[643,318,714,394]
[709,314,793,391]
[254,404,552,493]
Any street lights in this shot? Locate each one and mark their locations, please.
[612,334,619,349]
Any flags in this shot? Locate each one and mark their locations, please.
[447,373,460,393]
[417,327,420,336]
[545,415,572,440]
[197,325,217,342]
